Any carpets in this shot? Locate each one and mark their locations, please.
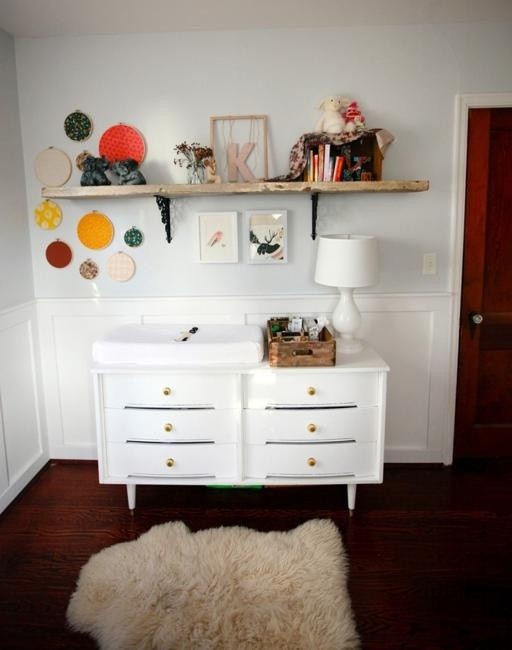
[66,520,361,649]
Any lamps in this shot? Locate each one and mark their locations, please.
[314,235,376,355]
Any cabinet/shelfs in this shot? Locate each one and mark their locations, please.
[89,365,390,517]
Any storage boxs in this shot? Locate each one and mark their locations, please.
[267,319,336,367]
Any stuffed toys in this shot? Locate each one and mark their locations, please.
[341,101,365,126]
[315,95,355,135]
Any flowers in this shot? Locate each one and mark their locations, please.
[173,141,216,185]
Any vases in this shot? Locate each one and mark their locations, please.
[185,166,208,185]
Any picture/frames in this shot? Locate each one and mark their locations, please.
[195,213,238,264]
[246,210,288,264]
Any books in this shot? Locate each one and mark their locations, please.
[301,142,346,182]
